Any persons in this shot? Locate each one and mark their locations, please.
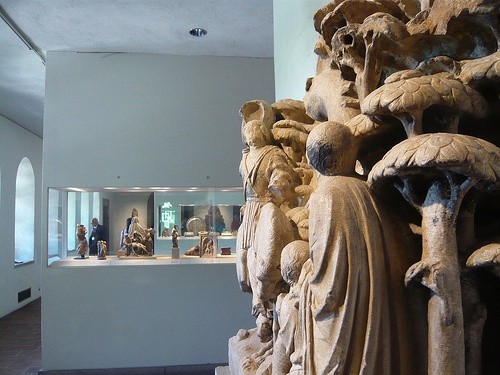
[123,217,224,258]
[89,218,107,255]
[120,218,139,254]
[227,1,423,375]
[204,206,226,233]
